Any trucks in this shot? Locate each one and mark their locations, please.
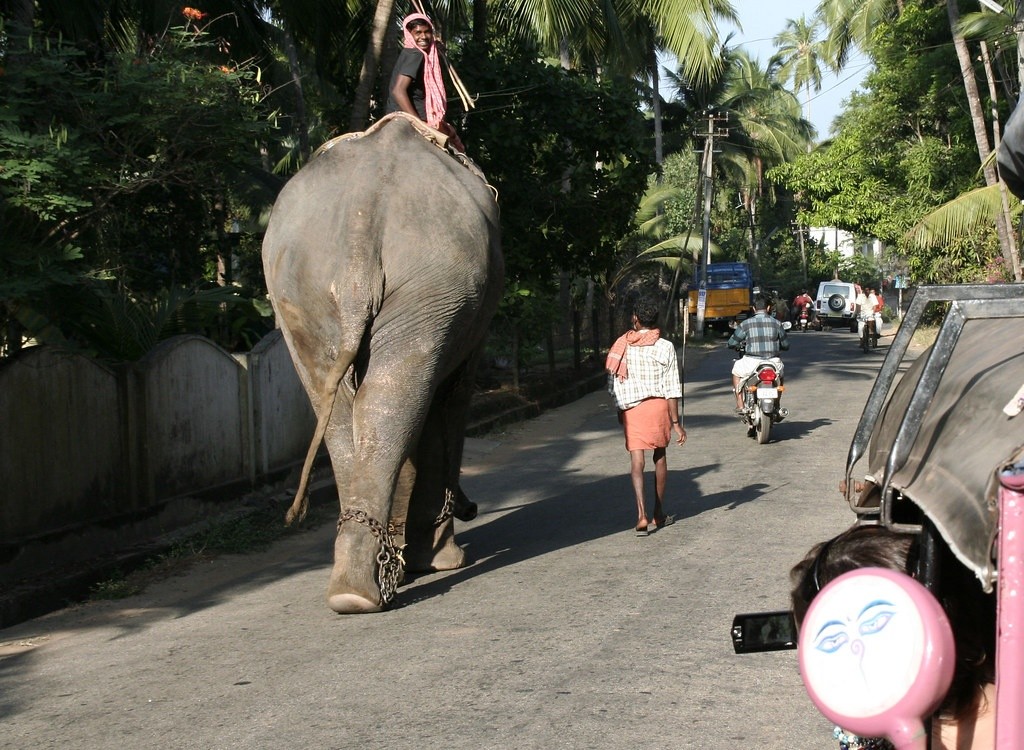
[688,262,754,337]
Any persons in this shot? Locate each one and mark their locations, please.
[868,288,885,338]
[385,13,469,157]
[856,285,880,349]
[606,299,687,538]
[773,286,816,331]
[725,291,790,413]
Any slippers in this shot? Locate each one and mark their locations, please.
[635,523,649,537]
[657,513,675,527]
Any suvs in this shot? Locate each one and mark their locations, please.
[812,279,859,331]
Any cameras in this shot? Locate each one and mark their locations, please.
[730,513,995,710]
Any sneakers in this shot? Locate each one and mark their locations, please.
[732,406,744,414]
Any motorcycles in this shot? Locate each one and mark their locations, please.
[857,312,875,353]
[730,322,790,444]
[796,304,809,332]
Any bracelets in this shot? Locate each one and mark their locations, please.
[673,420,680,425]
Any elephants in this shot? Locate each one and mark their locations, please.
[261,115,504,615]
